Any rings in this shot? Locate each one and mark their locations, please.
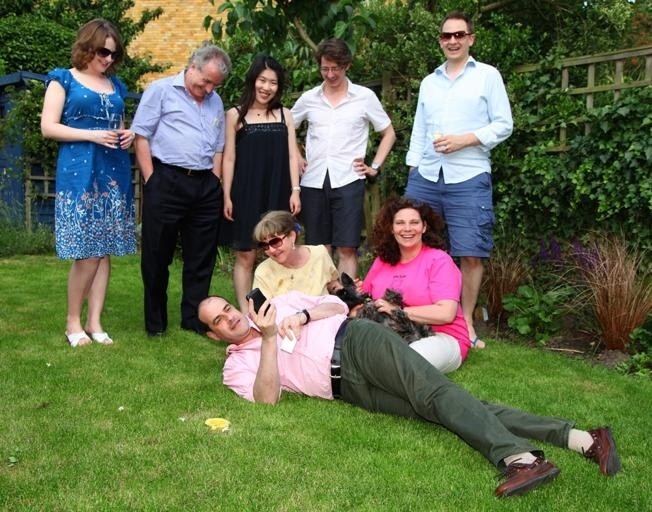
[380,301,383,306]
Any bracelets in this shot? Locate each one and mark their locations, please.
[290,186,301,192]
[297,309,311,325]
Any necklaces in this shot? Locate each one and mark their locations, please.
[254,110,267,117]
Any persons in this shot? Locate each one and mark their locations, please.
[196,289,621,497]
[222,53,301,312]
[252,210,342,300]
[131,46,231,338]
[291,37,397,284]
[405,13,513,350]
[41,18,135,347]
[327,198,471,373]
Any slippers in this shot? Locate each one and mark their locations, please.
[84,327,112,345]
[467,334,480,348]
[65,328,92,348]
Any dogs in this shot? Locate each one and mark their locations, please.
[326,272,434,344]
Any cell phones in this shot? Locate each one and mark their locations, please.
[246,288,271,317]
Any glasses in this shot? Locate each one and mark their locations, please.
[96,46,118,60]
[439,30,471,42]
[258,232,290,251]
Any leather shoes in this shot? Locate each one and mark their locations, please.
[582,426,622,476]
[494,456,561,499]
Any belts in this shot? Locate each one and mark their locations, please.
[172,164,208,177]
[330,319,351,400]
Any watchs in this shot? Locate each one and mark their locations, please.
[369,163,383,171]
